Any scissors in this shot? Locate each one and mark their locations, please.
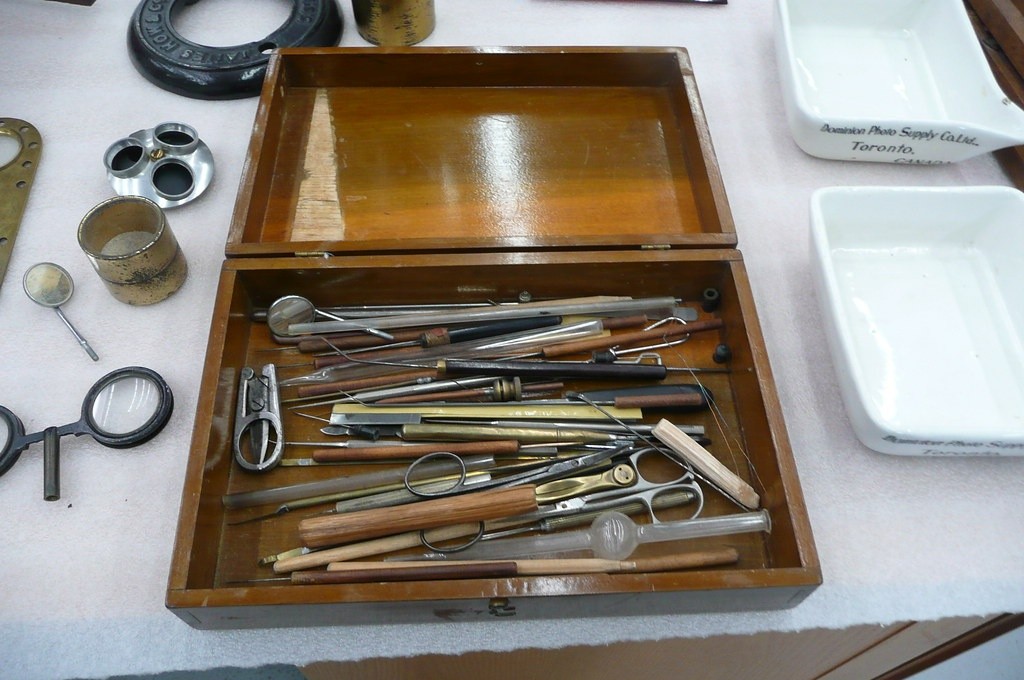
[481,447,705,532]
[405,442,636,553]
[230,363,285,472]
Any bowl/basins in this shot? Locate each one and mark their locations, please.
[771,0,1024,165]
[808,186,1024,456]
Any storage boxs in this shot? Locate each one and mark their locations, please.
[164,47,824,629]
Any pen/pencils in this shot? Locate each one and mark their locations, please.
[326,558,637,572]
[344,295,634,318]
